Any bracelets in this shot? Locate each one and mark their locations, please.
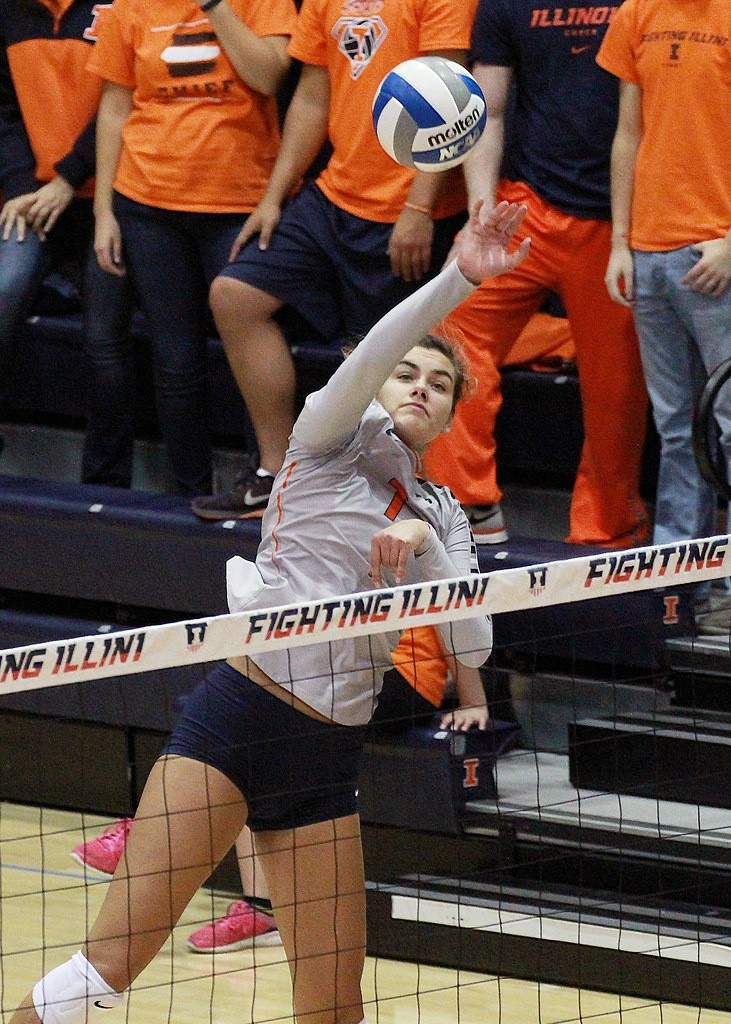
[199,0,223,12]
[402,200,434,216]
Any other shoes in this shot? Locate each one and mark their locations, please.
[695,590,731,633]
[461,502,508,544]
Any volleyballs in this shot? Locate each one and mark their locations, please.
[371,57,487,173]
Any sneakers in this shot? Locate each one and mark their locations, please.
[194,472,274,520]
[188,897,284,952]
[70,819,134,875]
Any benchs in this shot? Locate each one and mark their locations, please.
[0,208,657,895]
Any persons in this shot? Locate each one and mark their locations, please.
[11,200,530,1023]
[0,0,731,953]
